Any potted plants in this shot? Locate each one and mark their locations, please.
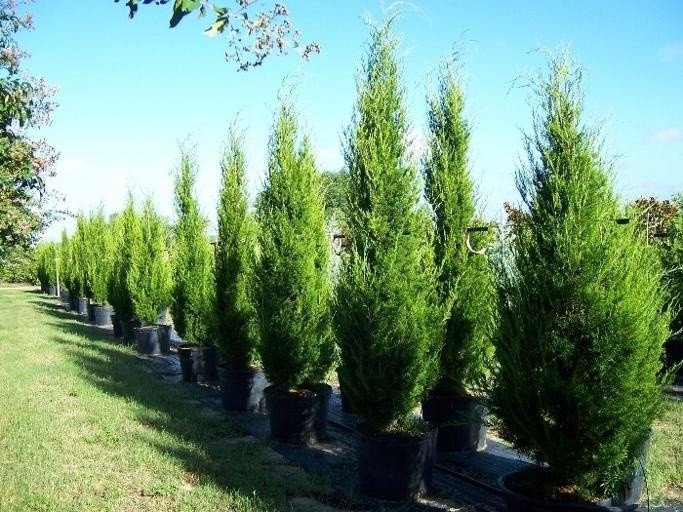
[206,105,254,410]
[167,127,210,382]
[453,42,681,512]
[330,3,426,505]
[245,67,320,444]
[297,131,334,442]
[37,189,172,355]
[203,239,224,384]
[422,49,500,456]
